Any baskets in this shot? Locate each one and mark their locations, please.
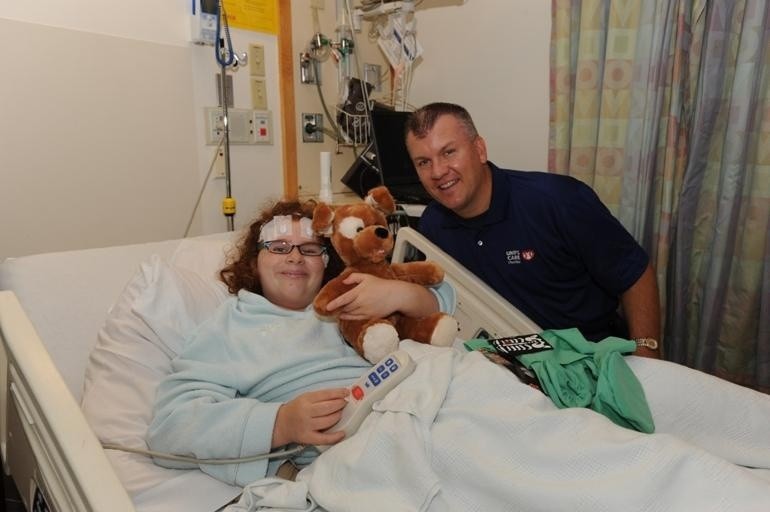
[335,97,373,146]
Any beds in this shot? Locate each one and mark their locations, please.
[2,227,769,510]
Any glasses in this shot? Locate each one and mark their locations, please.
[257,240,328,256]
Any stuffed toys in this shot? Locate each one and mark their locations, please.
[312,187,455,366]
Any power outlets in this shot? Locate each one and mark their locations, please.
[300,114,325,143]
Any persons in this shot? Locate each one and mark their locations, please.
[410,104,661,358]
[147,199,456,487]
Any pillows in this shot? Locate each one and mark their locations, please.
[84,257,223,462]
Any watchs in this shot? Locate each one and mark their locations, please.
[629,337,660,350]
